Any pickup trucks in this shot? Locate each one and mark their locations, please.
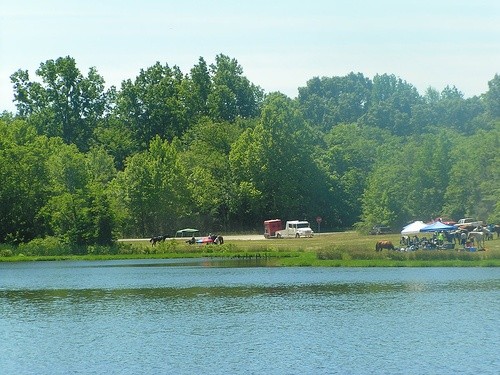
[275,220,314,238]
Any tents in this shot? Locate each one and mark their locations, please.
[420,221,458,249]
[401,221,431,248]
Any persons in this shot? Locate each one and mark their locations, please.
[461,231,467,246]
[413,235,418,247]
[482,226,490,241]
[439,233,444,245]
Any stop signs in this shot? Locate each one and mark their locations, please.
[317,217,322,223]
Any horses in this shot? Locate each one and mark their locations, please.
[150,232,170,247]
[468,232,484,248]
[376,240,394,252]
[471,227,490,241]
